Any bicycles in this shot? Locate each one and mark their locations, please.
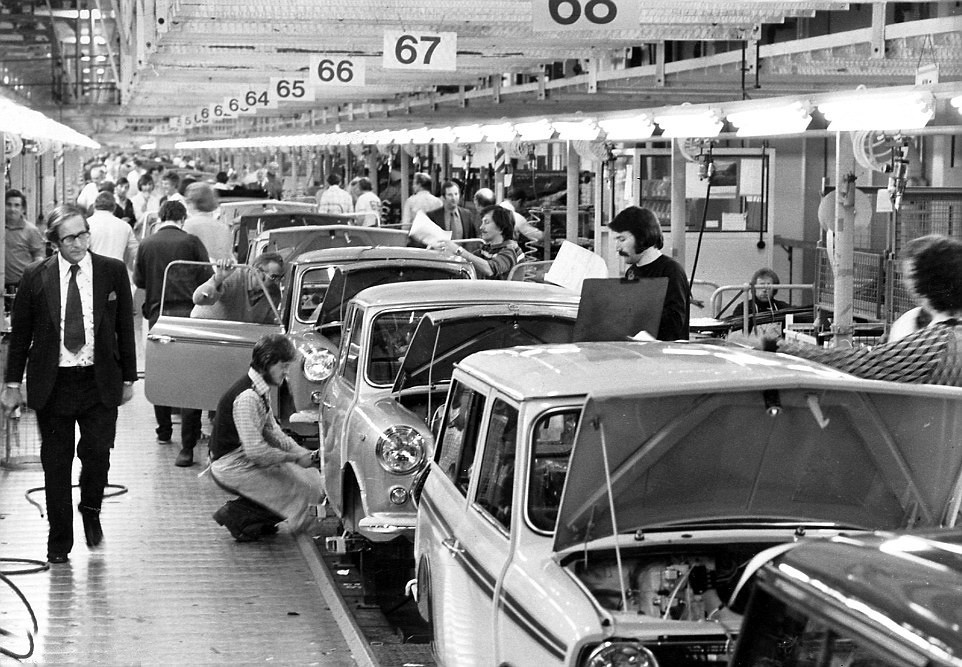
[0,291,29,461]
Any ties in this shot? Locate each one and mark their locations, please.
[64,264,86,353]
[450,213,456,241]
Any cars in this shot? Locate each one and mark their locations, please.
[309,275,677,601]
[401,339,962,667]
[144,168,477,441]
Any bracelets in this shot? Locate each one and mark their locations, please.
[6,385,21,389]
[124,381,133,385]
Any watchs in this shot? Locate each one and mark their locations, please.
[456,246,463,256]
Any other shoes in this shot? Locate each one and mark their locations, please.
[158,435,171,444]
[79,506,103,548]
[212,504,246,538]
[171,414,182,424]
[197,432,210,443]
[239,525,280,543]
[47,551,68,563]
[175,449,193,467]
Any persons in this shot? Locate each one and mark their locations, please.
[0,205,137,564]
[608,206,690,341]
[216,159,465,229]
[734,268,791,338]
[132,201,216,446]
[407,180,478,249]
[473,188,496,221]
[318,174,354,215]
[206,334,323,542]
[887,234,946,344]
[401,172,443,230]
[3,150,234,407]
[176,253,284,467]
[437,205,525,281]
[760,237,962,388]
[354,178,382,227]
[499,187,544,242]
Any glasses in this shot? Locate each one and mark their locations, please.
[262,269,284,282]
[57,229,91,247]
